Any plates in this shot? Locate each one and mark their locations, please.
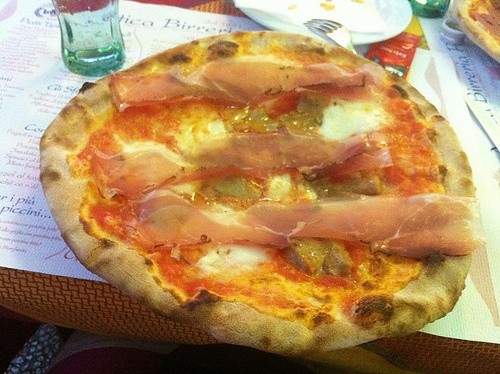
[233,1,413,47]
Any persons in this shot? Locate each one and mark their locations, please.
[47,328,318,374]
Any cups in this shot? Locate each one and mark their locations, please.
[409,0,451,18]
[51,0,127,77]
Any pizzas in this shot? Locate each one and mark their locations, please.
[38,31,481,359]
[453,0,500,63]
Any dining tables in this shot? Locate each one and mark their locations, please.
[0,0,499,374]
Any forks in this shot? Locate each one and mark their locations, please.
[304,18,357,56]
